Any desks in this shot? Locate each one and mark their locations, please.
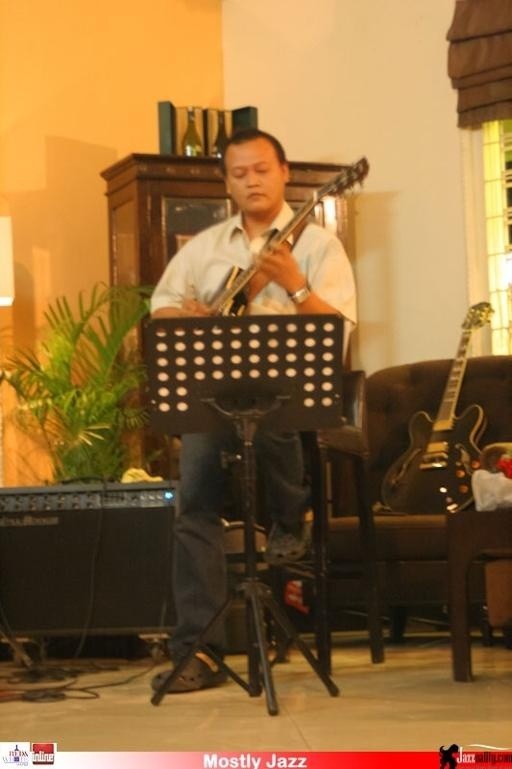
[443,510,509,681]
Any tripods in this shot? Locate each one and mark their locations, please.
[151,394,339,715]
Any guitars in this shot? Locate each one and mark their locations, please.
[377,299,495,517]
[204,156,368,320]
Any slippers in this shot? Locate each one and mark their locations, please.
[266,509,314,564]
[152,652,226,693]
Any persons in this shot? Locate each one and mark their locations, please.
[142,127,355,694]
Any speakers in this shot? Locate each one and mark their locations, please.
[0,481,178,641]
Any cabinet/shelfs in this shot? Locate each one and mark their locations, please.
[99,151,354,489]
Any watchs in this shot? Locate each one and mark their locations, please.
[286,279,319,306]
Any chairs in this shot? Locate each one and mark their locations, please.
[317,355,510,648]
